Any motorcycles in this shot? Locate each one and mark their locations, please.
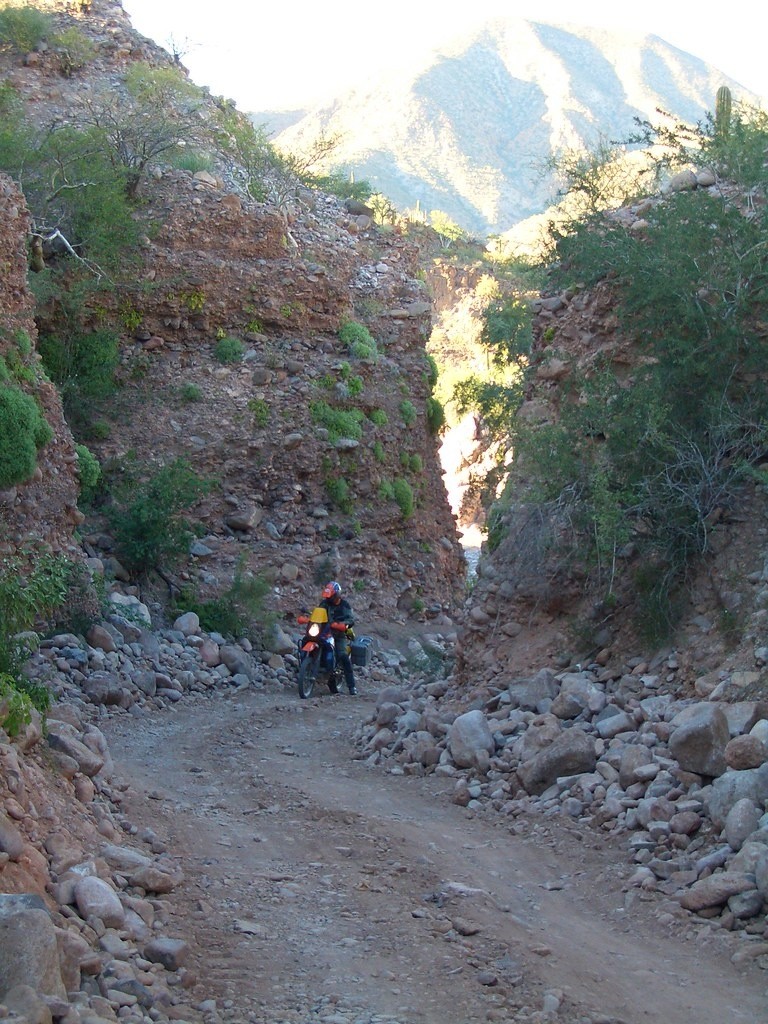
[294,606,354,698]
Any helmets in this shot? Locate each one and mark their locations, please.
[322,581,341,598]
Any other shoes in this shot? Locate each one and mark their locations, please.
[349,686,358,695]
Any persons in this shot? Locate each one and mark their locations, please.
[299,581,358,695]
